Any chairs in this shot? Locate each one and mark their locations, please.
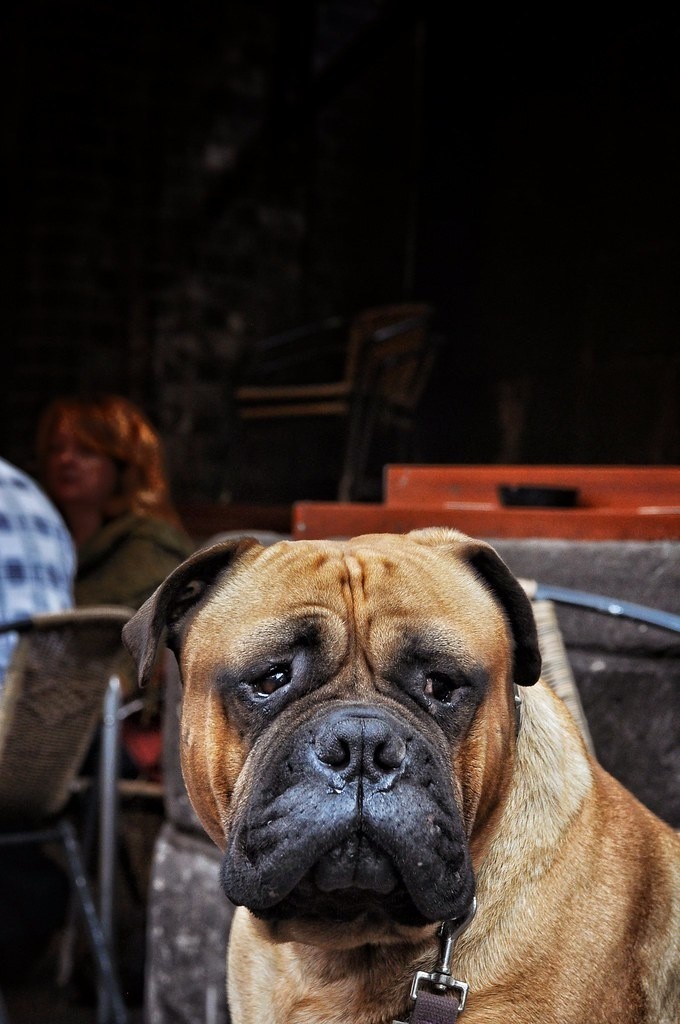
[217,297,449,500]
[0,607,132,1024]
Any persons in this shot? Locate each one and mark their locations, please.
[1,393,199,1012]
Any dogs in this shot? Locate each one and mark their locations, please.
[122,527,680,1024]
